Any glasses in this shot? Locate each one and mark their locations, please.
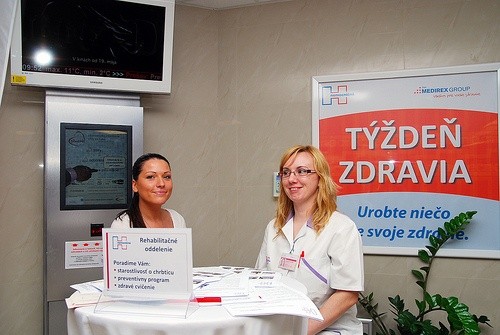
[278,170,317,178]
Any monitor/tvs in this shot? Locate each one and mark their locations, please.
[10,0,175,94]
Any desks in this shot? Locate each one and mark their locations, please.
[66,267,309,335]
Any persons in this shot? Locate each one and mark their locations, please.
[110,153,186,229]
[66,166,91,186]
[257,145,364,335]
